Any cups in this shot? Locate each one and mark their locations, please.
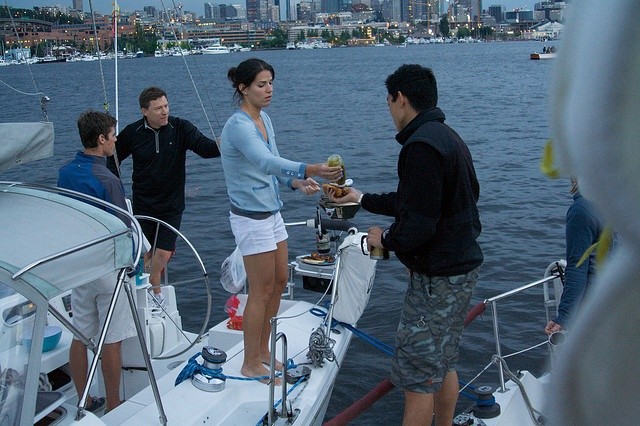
[361,232,391,260]
[547,332,570,354]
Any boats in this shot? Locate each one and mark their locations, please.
[228,43,251,52]
[530,51,556,59]
[200,44,230,55]
[321,255,568,426]
[1,120,377,426]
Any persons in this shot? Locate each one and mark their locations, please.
[551,46,555,53]
[542,44,547,53]
[324,63,484,426]
[545,176,620,337]
[58,110,139,414]
[108,87,221,292]
[546,47,551,53]
[220,56,343,386]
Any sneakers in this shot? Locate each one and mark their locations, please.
[152,297,166,315]
[83,395,107,413]
[104,400,126,415]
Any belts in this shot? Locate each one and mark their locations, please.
[230,202,273,220]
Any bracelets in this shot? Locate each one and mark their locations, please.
[358,193,366,207]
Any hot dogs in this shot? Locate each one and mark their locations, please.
[328,154,346,186]
[321,181,350,197]
